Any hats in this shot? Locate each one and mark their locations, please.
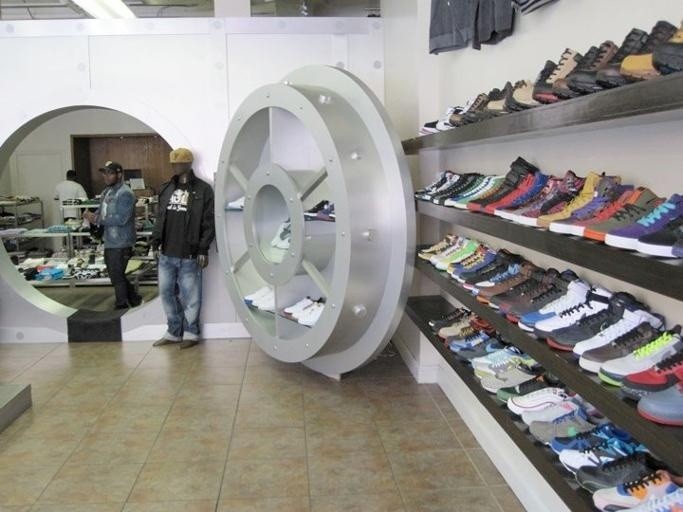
[99,160,122,173]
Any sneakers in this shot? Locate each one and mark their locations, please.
[488,267,545,310]
[423,107,463,134]
[672,239,683,258]
[304,201,329,218]
[480,174,526,212]
[467,156,536,212]
[636,213,683,258]
[575,451,674,495]
[522,398,596,426]
[448,325,496,353]
[298,297,325,326]
[414,171,476,209]
[451,249,497,280]
[437,311,481,339]
[487,82,512,115]
[114,302,131,309]
[449,93,487,127]
[551,423,632,456]
[457,249,510,283]
[534,285,613,339]
[457,334,512,359]
[517,278,591,333]
[584,186,666,241]
[579,321,681,374]
[292,299,317,318]
[443,176,494,207]
[436,98,476,132]
[571,189,634,237]
[463,254,520,291]
[558,437,650,476]
[153,337,182,345]
[506,387,584,416]
[454,175,504,209]
[552,41,618,98]
[513,179,562,223]
[598,331,683,387]
[432,310,471,335]
[181,341,198,348]
[475,260,536,304]
[653,42,683,75]
[480,362,546,394]
[537,171,621,229]
[418,106,459,137]
[435,240,479,271]
[471,344,521,369]
[440,175,485,205]
[621,347,683,401]
[472,262,519,296]
[592,470,683,512]
[430,236,471,265]
[566,28,649,93]
[596,21,677,88]
[276,236,291,249]
[474,352,538,380]
[475,83,513,119]
[244,286,275,311]
[271,219,291,247]
[499,269,560,314]
[317,204,335,220]
[283,296,313,313]
[496,374,565,404]
[501,175,556,219]
[506,270,579,324]
[615,487,683,512]
[428,306,466,327]
[636,381,683,427]
[519,171,586,228]
[620,18,683,82]
[417,233,458,260]
[546,292,649,352]
[529,404,621,446]
[465,88,499,123]
[604,194,683,250]
[512,49,583,107]
[505,60,557,111]
[485,173,535,215]
[486,79,525,114]
[549,177,634,234]
[447,243,489,274]
[132,300,144,307]
[445,319,489,346]
[227,196,245,209]
[572,308,665,359]
[493,171,550,217]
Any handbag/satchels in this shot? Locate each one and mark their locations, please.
[90,223,104,240]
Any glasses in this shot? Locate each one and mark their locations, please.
[104,170,114,175]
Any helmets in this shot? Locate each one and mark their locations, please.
[169,148,193,163]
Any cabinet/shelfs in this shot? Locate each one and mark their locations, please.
[69,224,159,288]
[0,200,45,265]
[16,229,71,288]
[60,198,159,251]
[402,72,683,512]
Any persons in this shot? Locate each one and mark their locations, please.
[53,170,89,248]
[92,166,107,197]
[83,161,144,309]
[149,149,214,350]
